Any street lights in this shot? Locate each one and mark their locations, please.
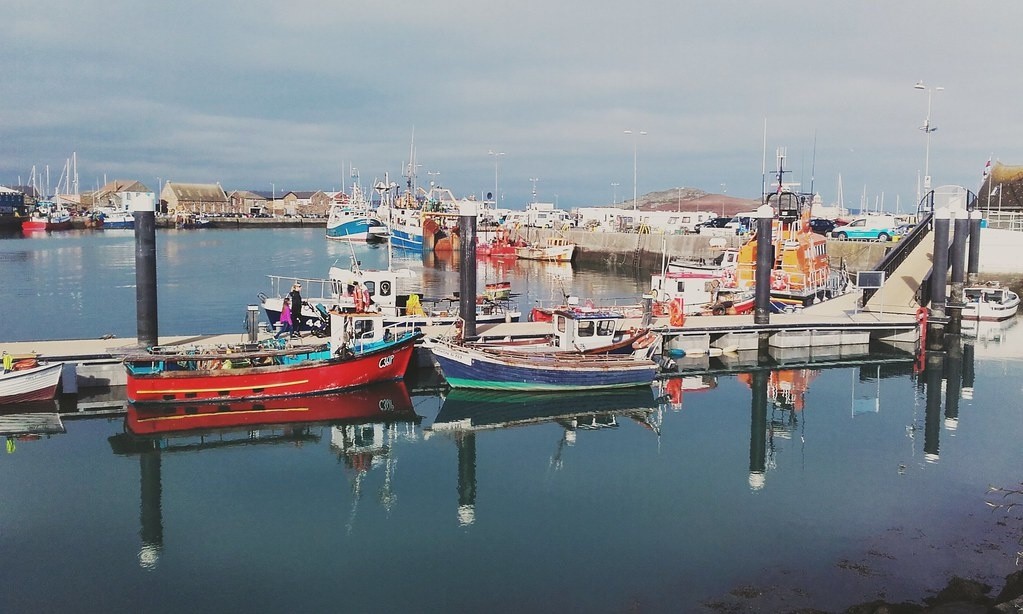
[530,178,539,203]
[676,187,685,212]
[624,130,648,232]
[488,150,506,210]
[353,167,360,190]
[611,182,621,208]
[913,82,948,214]
[720,183,727,218]
[156,176,163,214]
[269,182,276,219]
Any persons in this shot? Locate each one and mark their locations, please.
[289,282,303,338]
[274,298,294,339]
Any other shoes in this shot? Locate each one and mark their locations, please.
[298,335,303,338]
[274,334,279,339]
[290,334,298,339]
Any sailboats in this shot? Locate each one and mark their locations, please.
[19,150,136,232]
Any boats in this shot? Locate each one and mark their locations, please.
[419,307,671,391]
[0,349,65,404]
[123,304,424,404]
[961,279,1021,323]
[0,398,65,454]
[124,378,428,452]
[424,380,666,442]
[177,199,212,229]
[256,124,857,358]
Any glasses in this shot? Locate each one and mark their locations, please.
[297,286,301,288]
